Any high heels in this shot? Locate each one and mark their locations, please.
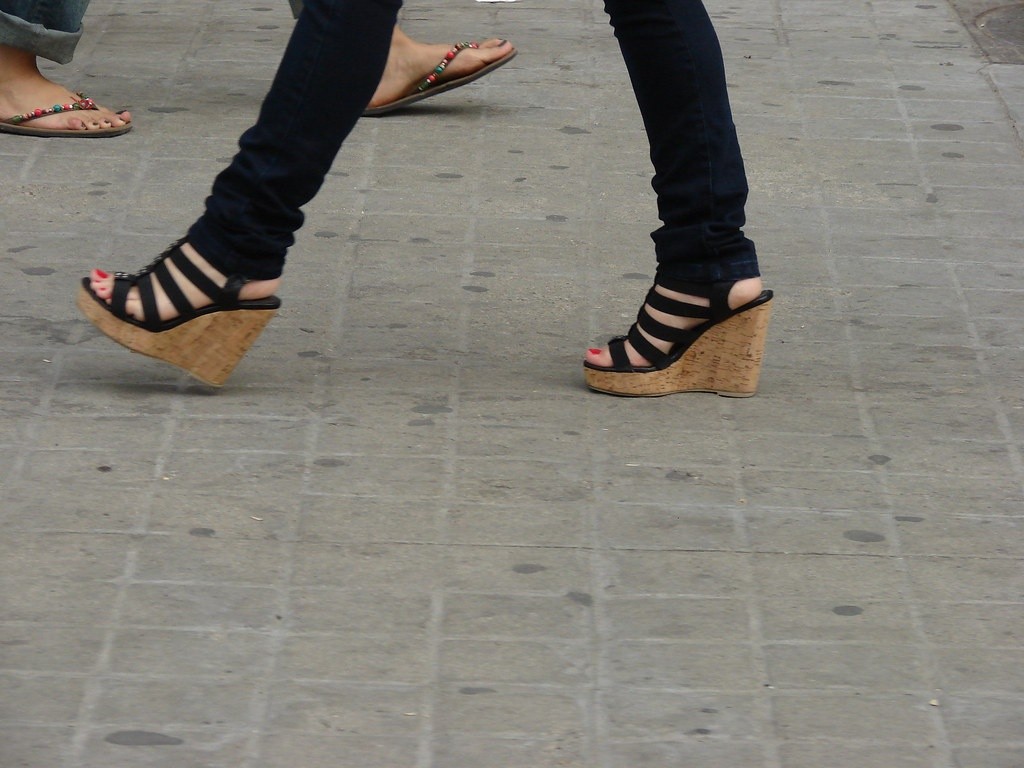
[583,272,774,398]
[76,233,282,387]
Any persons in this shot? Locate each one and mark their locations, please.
[77,0,774,398]
[0,0,518,138]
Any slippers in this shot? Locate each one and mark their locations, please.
[0,93,133,137]
[362,40,517,117]
